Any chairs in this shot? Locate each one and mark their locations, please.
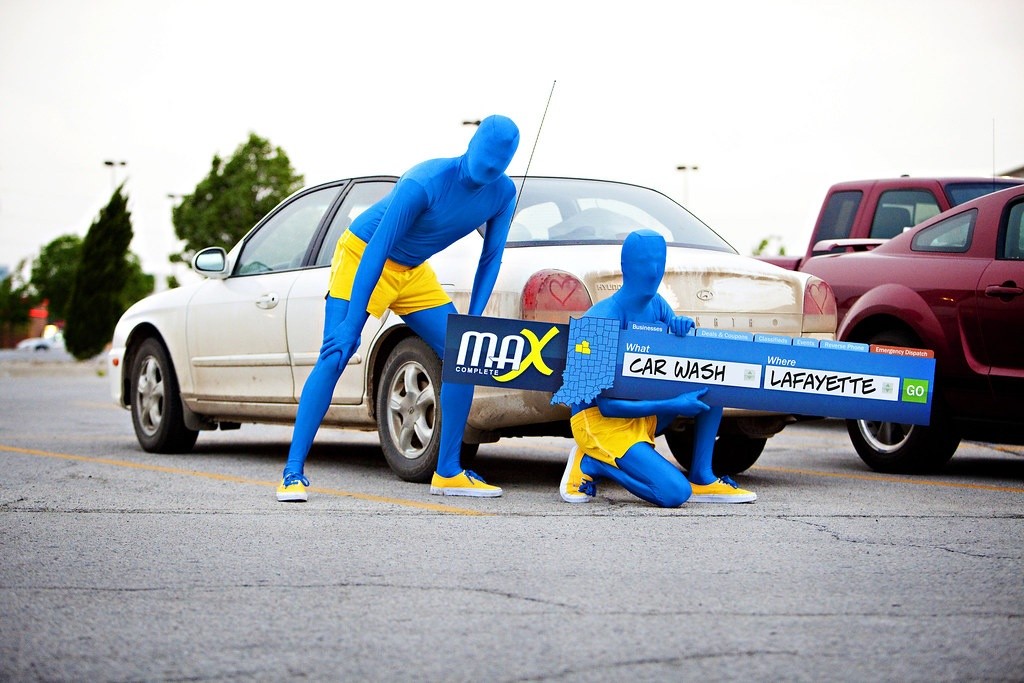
[870,206,911,239]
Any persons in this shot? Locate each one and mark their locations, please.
[277,115,523,503]
[560,229,758,509]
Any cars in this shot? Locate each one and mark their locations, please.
[16,333,64,353]
[799,181,1024,478]
[108,167,840,482]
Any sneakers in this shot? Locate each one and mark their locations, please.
[684,476,758,504]
[560,443,596,503]
[431,470,502,498]
[276,471,308,501]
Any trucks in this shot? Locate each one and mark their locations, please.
[756,173,1024,277]
